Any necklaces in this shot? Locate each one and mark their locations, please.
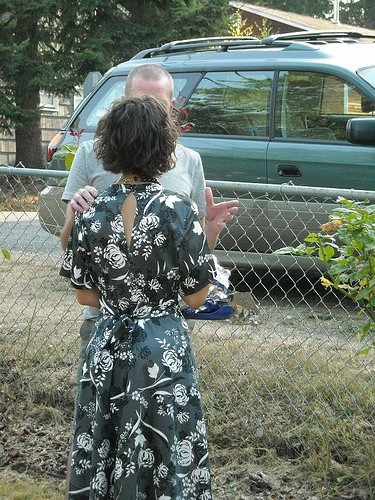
[113,173,161,185]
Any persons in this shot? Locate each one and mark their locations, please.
[59,66,240,500]
[59,97,220,499]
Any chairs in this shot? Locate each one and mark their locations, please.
[184,105,266,134]
[282,81,342,139]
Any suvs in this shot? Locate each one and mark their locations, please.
[37,31,374,280]
